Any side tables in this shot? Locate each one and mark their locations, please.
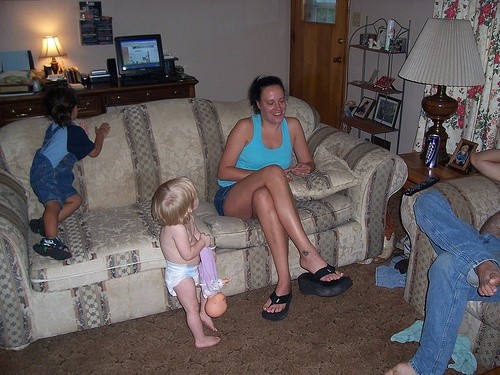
[399,152,467,188]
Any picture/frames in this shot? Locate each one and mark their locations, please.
[448,138,478,173]
[353,97,375,121]
[373,92,402,128]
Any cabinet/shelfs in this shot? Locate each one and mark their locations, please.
[0,73,199,127]
[341,17,411,155]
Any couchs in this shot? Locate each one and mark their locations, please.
[401,174,500,375]
[0,96,409,352]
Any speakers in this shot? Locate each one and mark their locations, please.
[107,58,118,81]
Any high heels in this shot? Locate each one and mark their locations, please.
[261,289,292,320]
[298,263,353,297]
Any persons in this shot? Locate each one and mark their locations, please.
[197,246,233,318]
[384,148,500,375]
[28,86,112,260]
[214,75,353,321]
[150,176,221,348]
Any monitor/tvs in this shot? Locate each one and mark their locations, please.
[114,34,165,76]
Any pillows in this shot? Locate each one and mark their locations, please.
[288,142,359,203]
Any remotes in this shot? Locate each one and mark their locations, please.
[404,177,440,196]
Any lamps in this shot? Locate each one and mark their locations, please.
[38,34,66,73]
[398,18,486,164]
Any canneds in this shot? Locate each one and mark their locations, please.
[423,134,440,169]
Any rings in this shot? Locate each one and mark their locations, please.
[303,174,304,175]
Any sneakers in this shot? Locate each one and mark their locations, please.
[29,217,46,238]
[33,238,72,260]
